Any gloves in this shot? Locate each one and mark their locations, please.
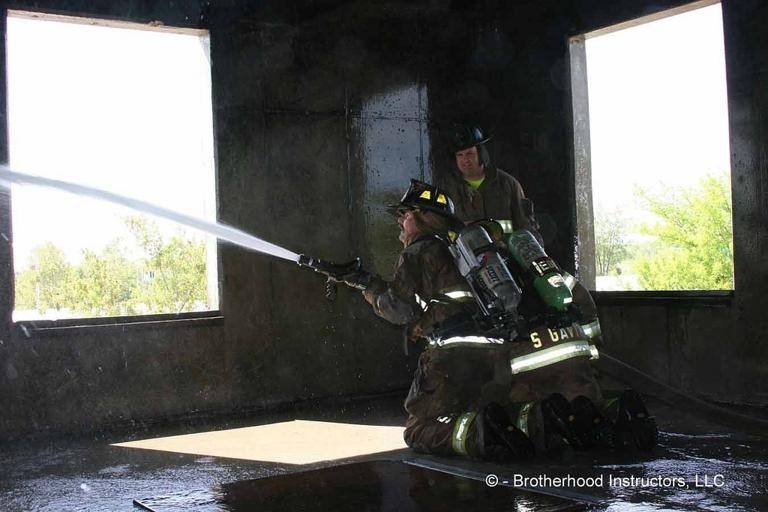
[362,275,384,304]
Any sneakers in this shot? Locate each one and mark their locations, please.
[547,389,662,451]
[485,402,536,463]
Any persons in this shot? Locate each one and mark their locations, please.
[364,119,658,461]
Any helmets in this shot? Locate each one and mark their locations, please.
[385,179,465,231]
[452,125,490,152]
